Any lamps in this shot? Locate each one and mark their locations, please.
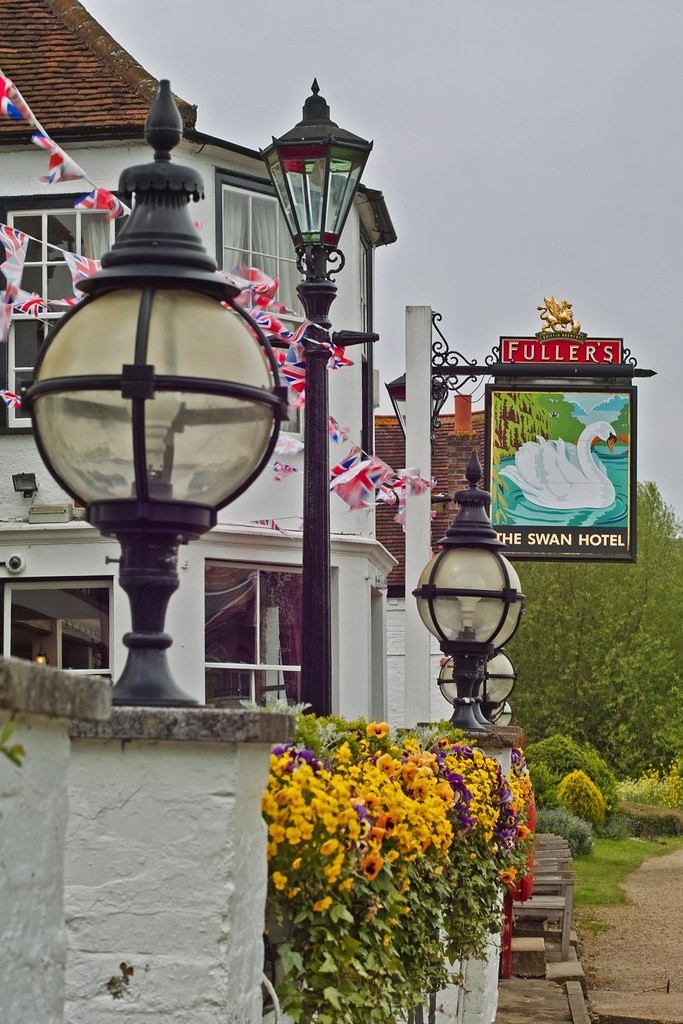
[409,451,524,736]
[11,76,292,713]
[10,472,38,498]
[34,642,49,666]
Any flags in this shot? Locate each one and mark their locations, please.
[0,67,438,543]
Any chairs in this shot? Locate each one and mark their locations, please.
[498,815,577,977]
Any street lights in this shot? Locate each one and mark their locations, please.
[257,73,378,727]
